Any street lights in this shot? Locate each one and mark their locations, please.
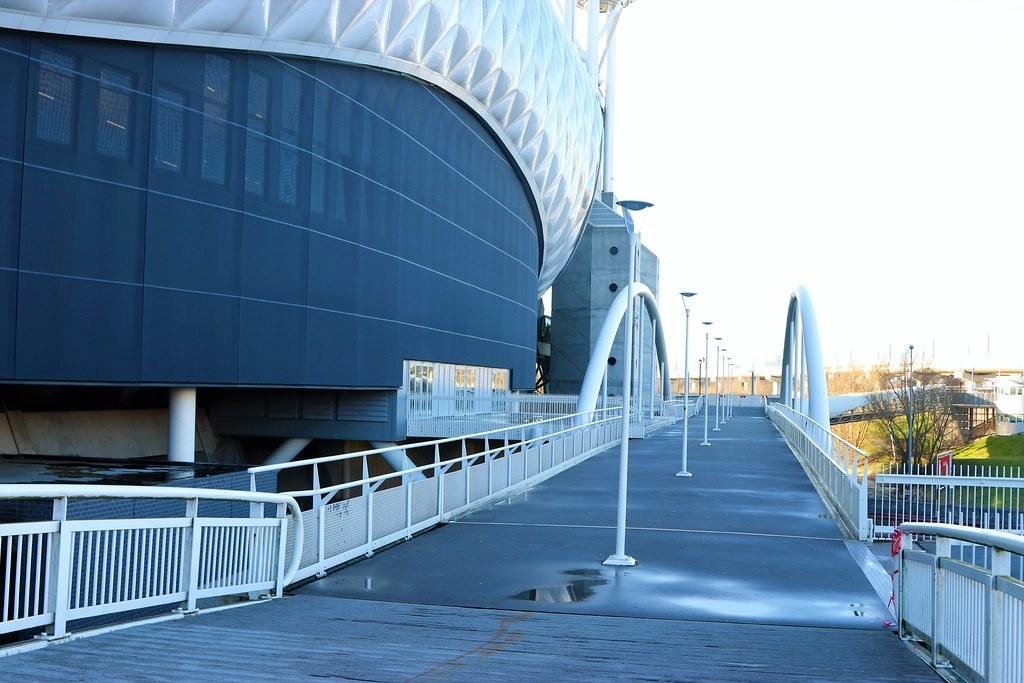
[674,292,698,478]
[601,200,655,566]
[700,321,734,446]
